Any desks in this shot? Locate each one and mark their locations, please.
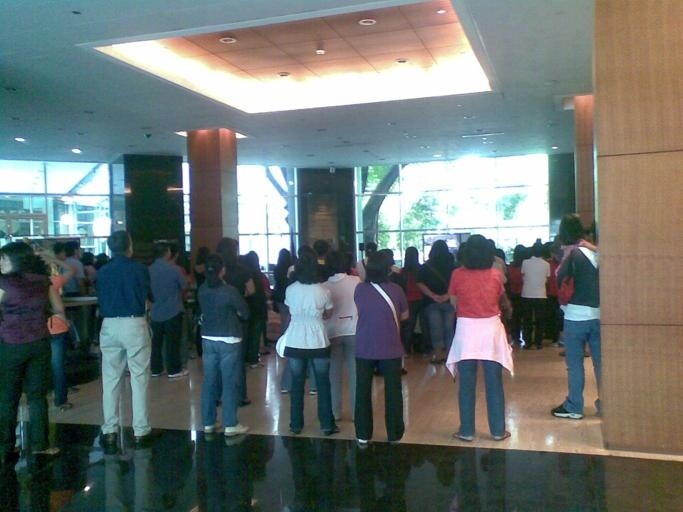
[64,297,98,365]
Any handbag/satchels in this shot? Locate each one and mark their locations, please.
[44,313,72,341]
[273,330,291,357]
[400,382,412,430]
[554,276,573,307]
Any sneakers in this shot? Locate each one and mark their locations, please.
[237,397,252,407]
[215,399,222,407]
[308,390,317,395]
[225,422,248,436]
[594,397,600,414]
[150,369,166,376]
[551,405,583,419]
[168,368,188,378]
[249,361,264,369]
[288,423,303,436]
[390,432,407,445]
[323,426,340,434]
[54,403,71,414]
[357,438,368,444]
[203,422,221,434]
[280,387,287,394]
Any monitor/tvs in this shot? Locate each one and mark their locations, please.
[423,234,470,262]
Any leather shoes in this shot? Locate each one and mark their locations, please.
[491,431,512,441]
[31,447,61,455]
[99,431,119,443]
[133,429,165,444]
[452,433,476,442]
[4,447,20,454]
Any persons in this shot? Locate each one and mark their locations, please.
[1,231,109,454]
[2,433,517,511]
[94,231,514,442]
[509,213,603,419]
